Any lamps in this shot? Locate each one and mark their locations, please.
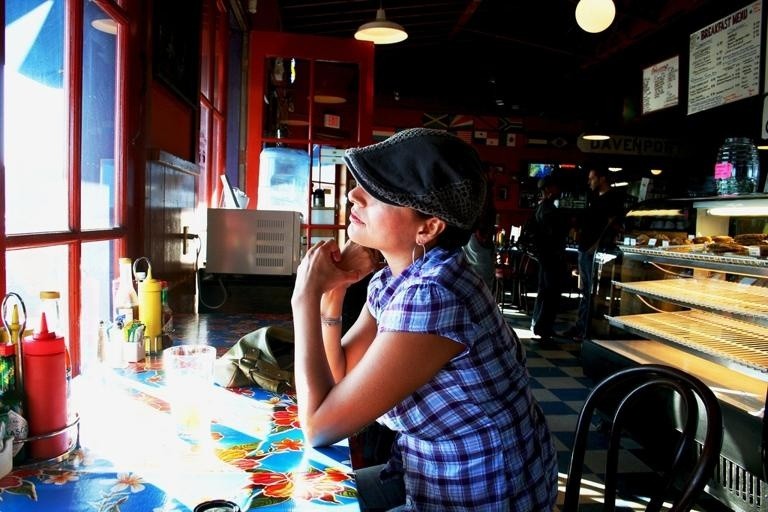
[353,0,409,45]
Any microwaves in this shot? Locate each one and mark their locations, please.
[205,207,302,276]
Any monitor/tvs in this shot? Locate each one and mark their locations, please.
[518,159,590,210]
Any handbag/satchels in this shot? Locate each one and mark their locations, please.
[516,218,543,263]
[212,323,295,394]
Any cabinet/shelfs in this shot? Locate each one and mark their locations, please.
[580,191,768,511]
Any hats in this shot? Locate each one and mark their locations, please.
[344,127,488,232]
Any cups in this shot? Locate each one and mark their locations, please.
[162,345,216,442]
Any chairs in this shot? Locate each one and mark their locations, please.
[493,236,535,317]
[555,363,725,512]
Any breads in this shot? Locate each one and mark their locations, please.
[636,230,768,259]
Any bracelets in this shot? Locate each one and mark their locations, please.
[319,312,344,327]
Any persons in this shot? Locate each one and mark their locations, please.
[562,165,625,343]
[525,174,567,337]
[291,126,561,511]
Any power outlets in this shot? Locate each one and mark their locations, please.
[184,226,191,255]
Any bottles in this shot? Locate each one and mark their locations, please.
[0,292,69,461]
[114,257,169,357]
[313,189,325,207]
[715,138,761,195]
